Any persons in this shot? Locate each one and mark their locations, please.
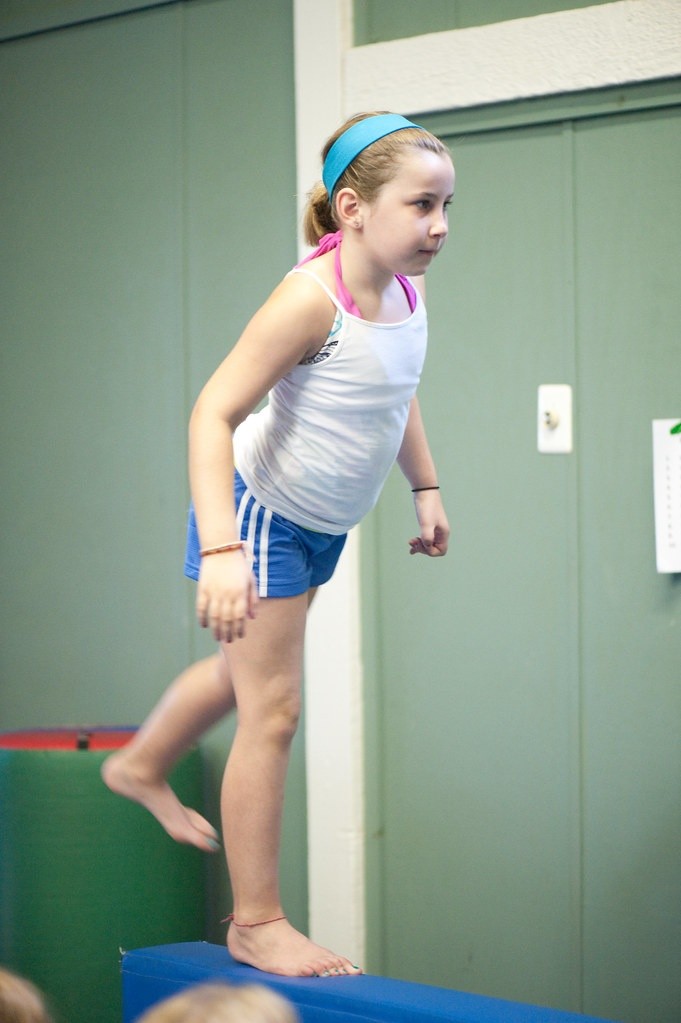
[103,113,458,977]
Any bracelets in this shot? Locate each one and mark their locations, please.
[199,540,255,573]
[410,486,442,492]
